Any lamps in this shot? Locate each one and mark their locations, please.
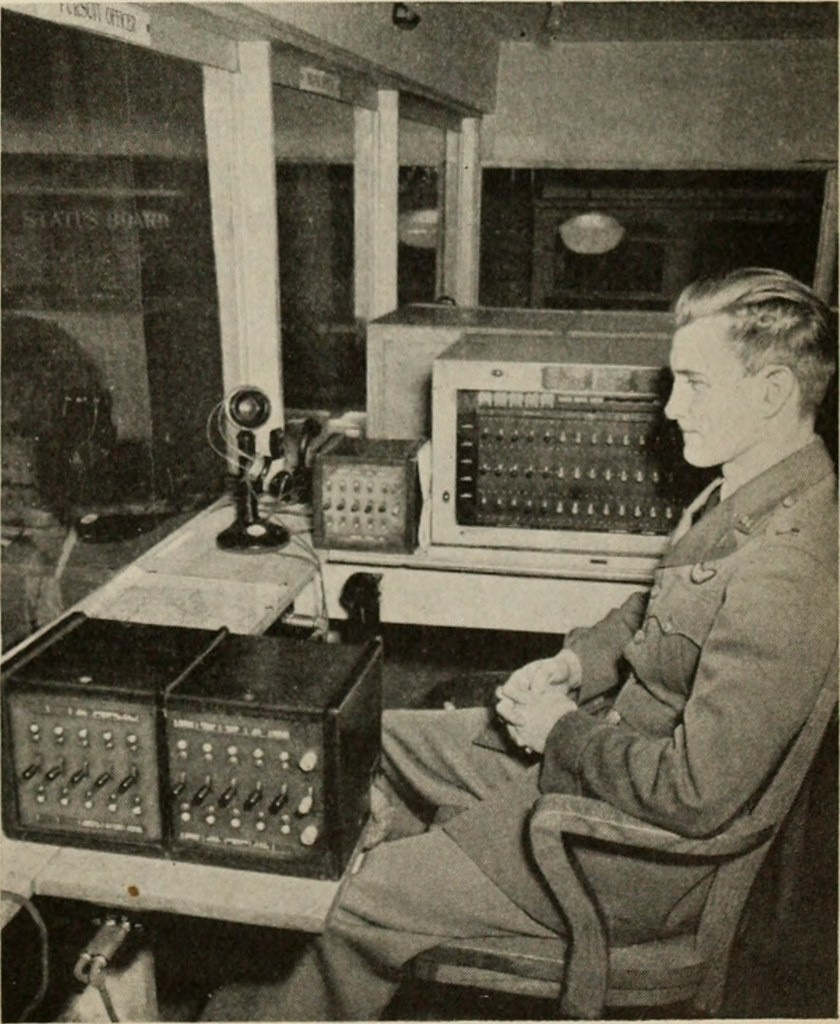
[558,170,626,256]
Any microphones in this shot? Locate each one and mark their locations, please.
[215,384,291,554]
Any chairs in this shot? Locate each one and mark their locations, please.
[413,639,836,1022]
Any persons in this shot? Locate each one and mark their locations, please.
[192,267,838,1015]
